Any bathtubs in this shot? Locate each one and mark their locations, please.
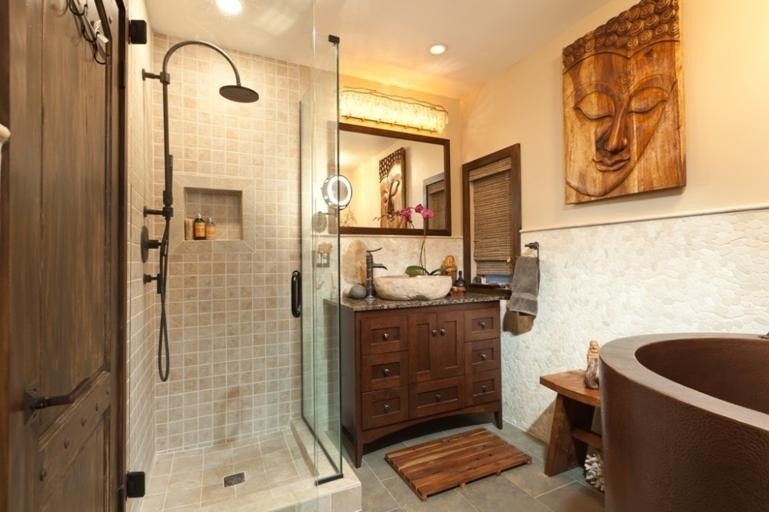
[597,331,769,511]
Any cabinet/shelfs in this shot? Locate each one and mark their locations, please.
[325,301,503,469]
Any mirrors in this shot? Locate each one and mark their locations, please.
[326,120,453,234]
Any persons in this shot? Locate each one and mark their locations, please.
[563,0,679,197]
[379,148,406,228]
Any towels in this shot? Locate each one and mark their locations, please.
[507,255,542,316]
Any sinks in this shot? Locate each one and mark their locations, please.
[372,274,453,301]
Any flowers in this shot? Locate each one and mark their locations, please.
[408,205,446,274]
[395,209,414,228]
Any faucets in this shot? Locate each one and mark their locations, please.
[366,246,388,300]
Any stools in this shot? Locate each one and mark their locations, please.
[539,365,604,478]
